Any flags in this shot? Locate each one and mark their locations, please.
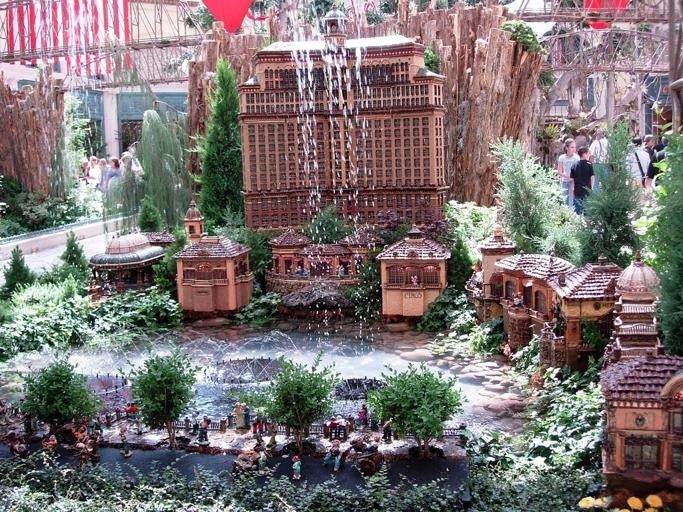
[6,0,68,65]
[65,1,131,76]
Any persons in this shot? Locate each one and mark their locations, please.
[323,404,394,444]
[94,403,139,455]
[655,136,668,160]
[15,412,96,466]
[557,137,580,208]
[569,145,595,216]
[227,402,277,439]
[511,291,524,308]
[622,136,651,193]
[183,415,226,442]
[645,134,659,190]
[237,435,301,473]
[83,154,121,192]
[550,316,561,337]
[576,125,610,197]
[286,423,310,438]
[323,439,341,471]
